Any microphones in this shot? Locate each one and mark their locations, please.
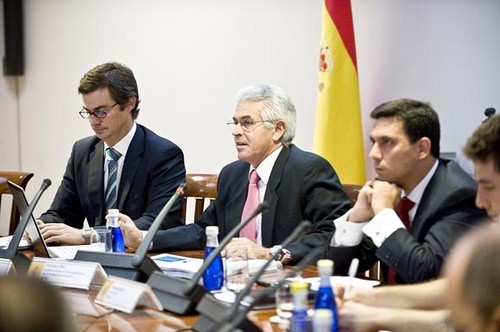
[73,181,188,285]
[0,178,51,278]
[145,201,270,316]
[216,246,325,332]
[191,219,312,332]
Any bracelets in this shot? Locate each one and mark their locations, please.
[270,247,281,261]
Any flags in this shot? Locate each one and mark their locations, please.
[315,0,367,186]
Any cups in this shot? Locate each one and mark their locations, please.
[275,268,303,320]
[88,229,113,253]
[223,245,250,292]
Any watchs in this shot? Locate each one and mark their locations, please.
[82,228,96,244]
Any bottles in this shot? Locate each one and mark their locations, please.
[201,224,224,290]
[290,282,312,332]
[309,258,339,332]
[105,209,126,254]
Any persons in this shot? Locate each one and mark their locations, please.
[105,84,352,267]
[24,62,185,245]
[333,114,500,332]
[325,99,488,283]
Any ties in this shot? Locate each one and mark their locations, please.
[104,147,121,216]
[239,169,261,242]
[387,196,415,286]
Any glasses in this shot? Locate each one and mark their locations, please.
[227,118,276,131]
[79,102,120,119]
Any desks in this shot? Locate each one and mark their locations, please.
[0,236,379,332]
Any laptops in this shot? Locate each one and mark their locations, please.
[3,182,105,261]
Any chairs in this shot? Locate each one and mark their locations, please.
[184,172,219,227]
[0,171,35,236]
[343,182,377,280]
